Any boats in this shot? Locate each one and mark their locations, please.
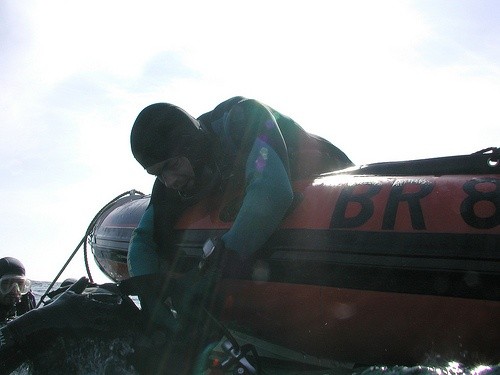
[91,147,500,375]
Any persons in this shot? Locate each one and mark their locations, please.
[126,95,356,333]
[0,257,36,328]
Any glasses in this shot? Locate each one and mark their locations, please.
[2,273,31,296]
